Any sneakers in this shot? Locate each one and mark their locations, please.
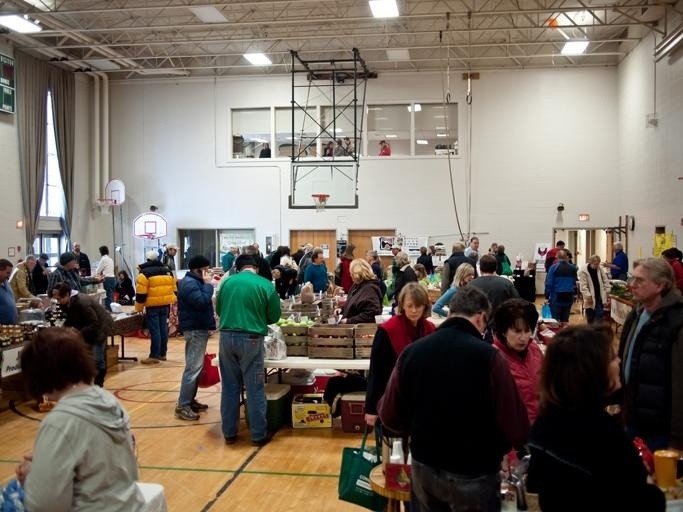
[226,437,236,444]
[141,355,166,363]
[252,433,271,446]
[174,406,200,420]
[188,400,208,409]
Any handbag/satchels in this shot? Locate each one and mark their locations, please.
[542,304,552,318]
[197,354,220,388]
[502,261,512,275]
[335,258,341,278]
[338,447,388,512]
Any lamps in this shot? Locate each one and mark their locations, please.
[189,6,227,24]
[242,53,273,67]
[561,37,592,56]
[386,49,409,61]
[369,0,400,18]
[2,14,42,34]
[16,220,23,229]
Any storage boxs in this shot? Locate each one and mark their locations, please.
[103,344,120,368]
[243,292,378,434]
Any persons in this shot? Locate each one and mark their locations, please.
[258,142,271,158]
[16,326,146,512]
[376,140,391,156]
[338,238,683,512]
[343,137,353,156]
[333,138,345,156]
[1,242,117,387]
[117,240,330,365]
[214,253,281,445]
[174,255,216,421]
[323,141,333,157]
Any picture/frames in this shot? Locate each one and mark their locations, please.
[8,247,15,256]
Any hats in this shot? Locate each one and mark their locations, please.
[367,251,378,256]
[145,250,159,260]
[25,256,36,264]
[40,253,49,259]
[390,244,401,251]
[167,243,180,249]
[189,255,209,269]
[302,244,314,250]
[60,253,75,266]
[237,256,258,269]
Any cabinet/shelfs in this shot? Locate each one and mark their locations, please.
[608,293,634,333]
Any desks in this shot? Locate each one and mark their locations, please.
[0,311,146,380]
[368,463,412,512]
